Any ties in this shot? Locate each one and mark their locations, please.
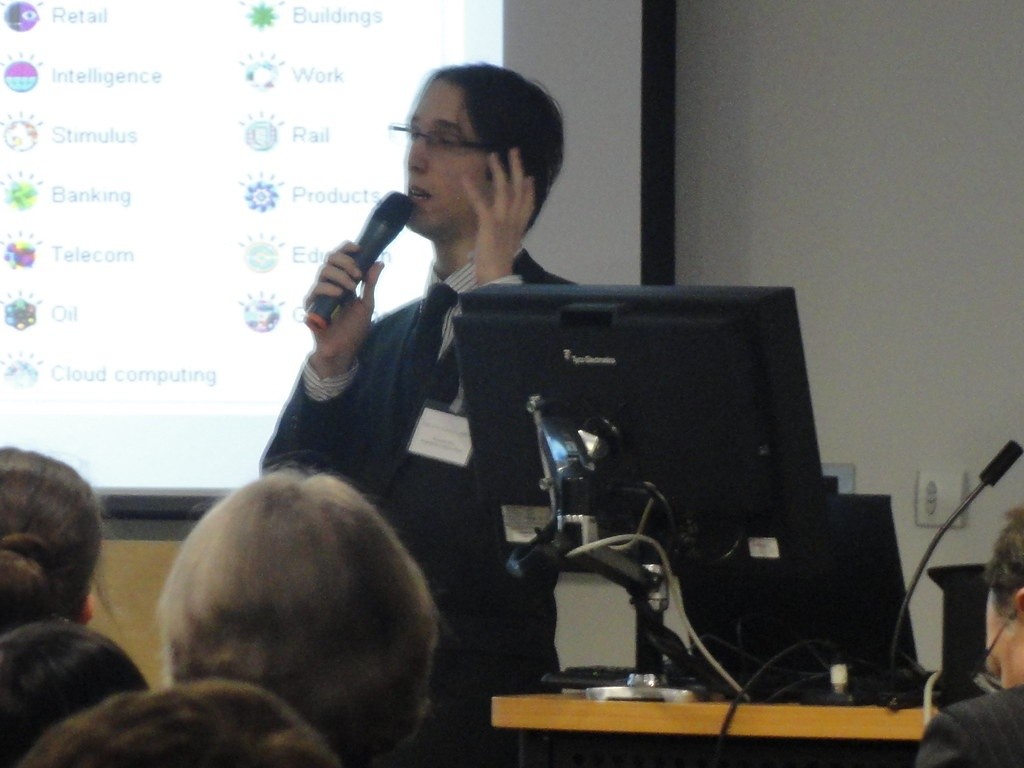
[357,282,460,504]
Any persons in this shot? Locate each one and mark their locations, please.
[915,507,1024,768]
[159,467,439,768]
[262,64,580,620]
[0,621,150,768]
[0,448,104,625]
[16,678,341,768]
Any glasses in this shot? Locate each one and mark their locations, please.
[968,607,1017,696]
[388,121,497,156]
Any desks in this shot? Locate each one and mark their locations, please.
[491,689,934,746]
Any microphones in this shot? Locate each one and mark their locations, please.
[875,440,1024,708]
[304,191,414,332]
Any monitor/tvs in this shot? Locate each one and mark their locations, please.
[450,283,849,636]
